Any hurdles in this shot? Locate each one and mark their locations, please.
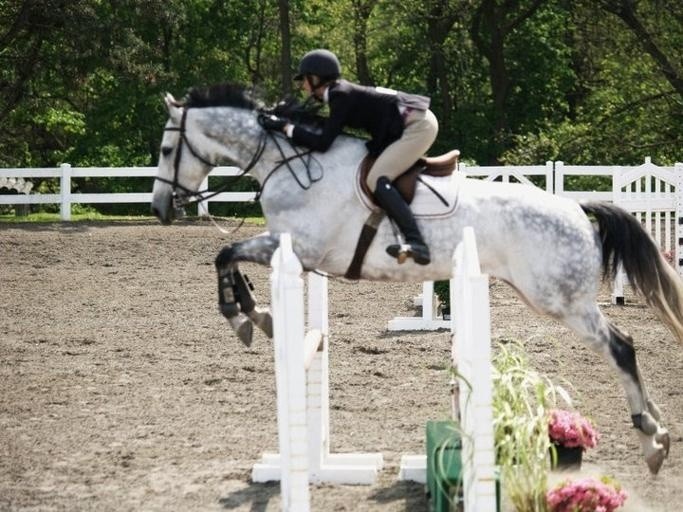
[253,223,385,508]
[388,226,526,507]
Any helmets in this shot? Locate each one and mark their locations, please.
[293,48,342,81]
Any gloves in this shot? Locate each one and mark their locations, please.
[262,114,289,133]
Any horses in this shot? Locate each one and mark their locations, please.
[149,91,683,477]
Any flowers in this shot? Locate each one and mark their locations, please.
[543,475,629,512]
[547,407,599,449]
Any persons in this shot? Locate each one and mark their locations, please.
[260,47,440,265]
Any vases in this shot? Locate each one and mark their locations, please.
[554,441,584,464]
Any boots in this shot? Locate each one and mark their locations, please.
[373,174,431,265]
